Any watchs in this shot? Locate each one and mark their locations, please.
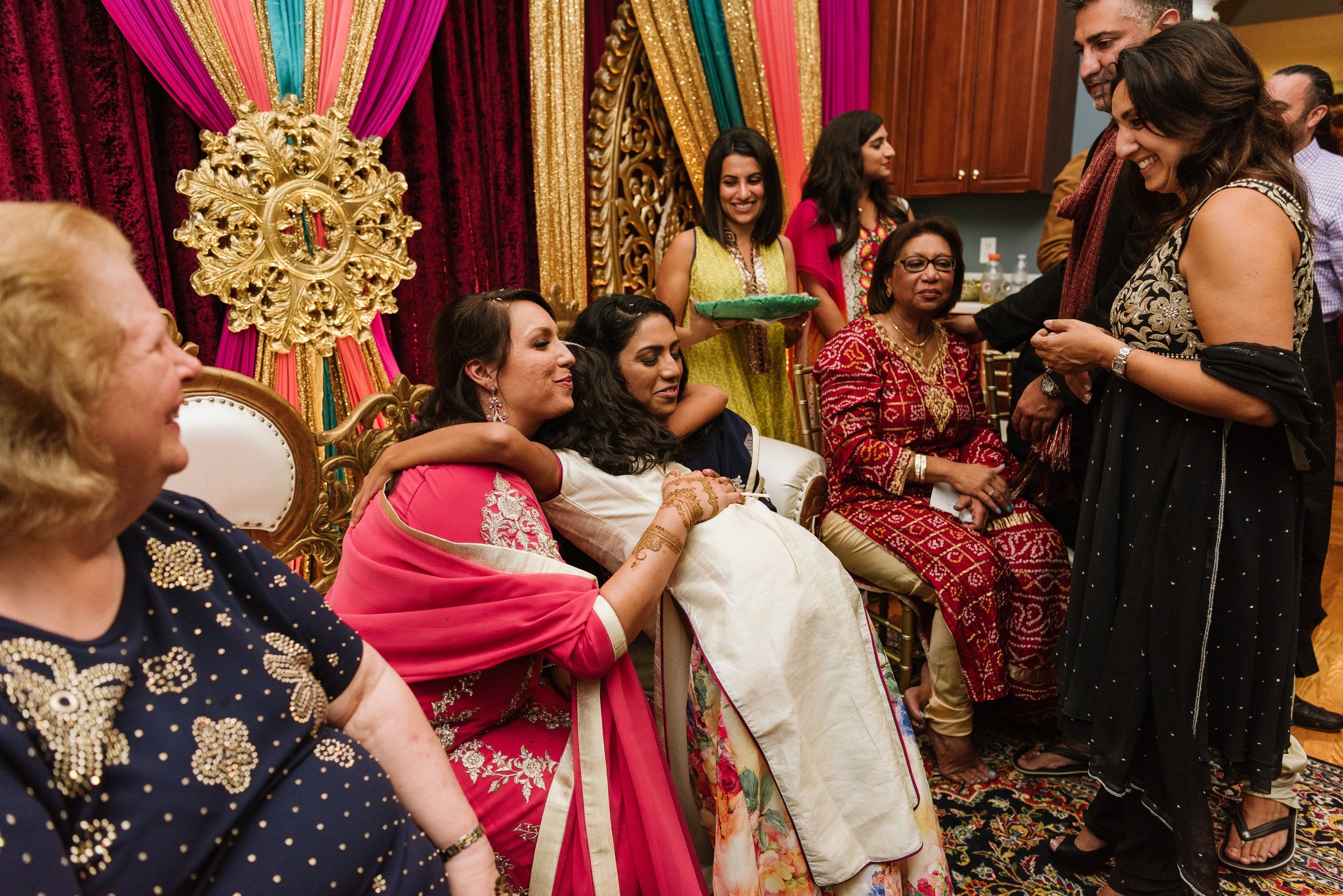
[1111,344,1138,381]
[1041,372,1060,398]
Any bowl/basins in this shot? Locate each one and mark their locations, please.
[959,279,1010,302]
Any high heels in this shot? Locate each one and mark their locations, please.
[1046,833,1115,873]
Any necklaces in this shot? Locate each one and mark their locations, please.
[887,309,932,362]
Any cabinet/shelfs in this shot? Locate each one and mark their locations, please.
[872,0,1080,200]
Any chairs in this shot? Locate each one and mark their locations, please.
[792,364,934,695]
[158,306,435,601]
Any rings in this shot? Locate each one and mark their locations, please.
[1045,361,1054,374]
[988,489,995,496]
[713,319,722,330]
[788,322,792,327]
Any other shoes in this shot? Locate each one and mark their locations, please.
[1292,694,1343,732]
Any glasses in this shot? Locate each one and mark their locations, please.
[894,256,956,273]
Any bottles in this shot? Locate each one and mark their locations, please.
[979,253,1005,304]
[1010,254,1029,295]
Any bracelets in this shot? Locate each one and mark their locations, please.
[914,454,921,482]
[439,823,485,863]
[919,454,926,484]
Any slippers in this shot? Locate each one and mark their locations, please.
[1217,801,1298,874]
[1010,738,1091,775]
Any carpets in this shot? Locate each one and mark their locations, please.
[915,728,1343,896]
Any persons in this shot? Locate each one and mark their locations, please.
[785,111,916,387]
[1261,63,1342,730]
[940,1,1302,871]
[2,200,499,896]
[564,291,775,515]
[657,129,808,445]
[1028,18,1338,896]
[325,283,747,896]
[810,218,1070,787]
[347,349,949,895]
